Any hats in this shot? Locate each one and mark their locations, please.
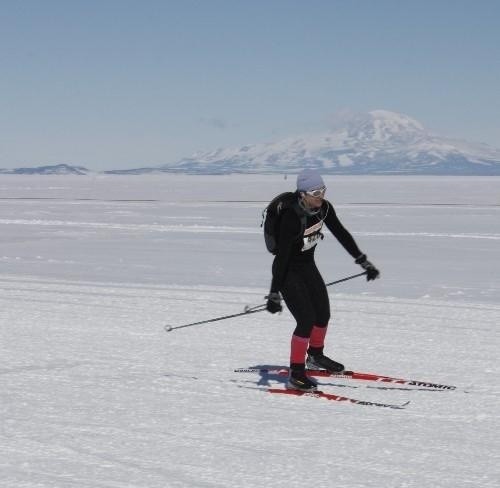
[296,170,324,192]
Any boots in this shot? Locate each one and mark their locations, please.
[308,347,344,371]
[290,363,316,389]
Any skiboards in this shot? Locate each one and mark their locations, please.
[161,367,469,409]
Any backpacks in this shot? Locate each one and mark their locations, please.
[264,192,326,254]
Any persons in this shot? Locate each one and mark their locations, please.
[266,167,378,390]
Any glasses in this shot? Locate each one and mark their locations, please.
[307,188,326,196]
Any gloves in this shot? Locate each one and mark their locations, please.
[264,294,282,312]
[356,255,378,281]
